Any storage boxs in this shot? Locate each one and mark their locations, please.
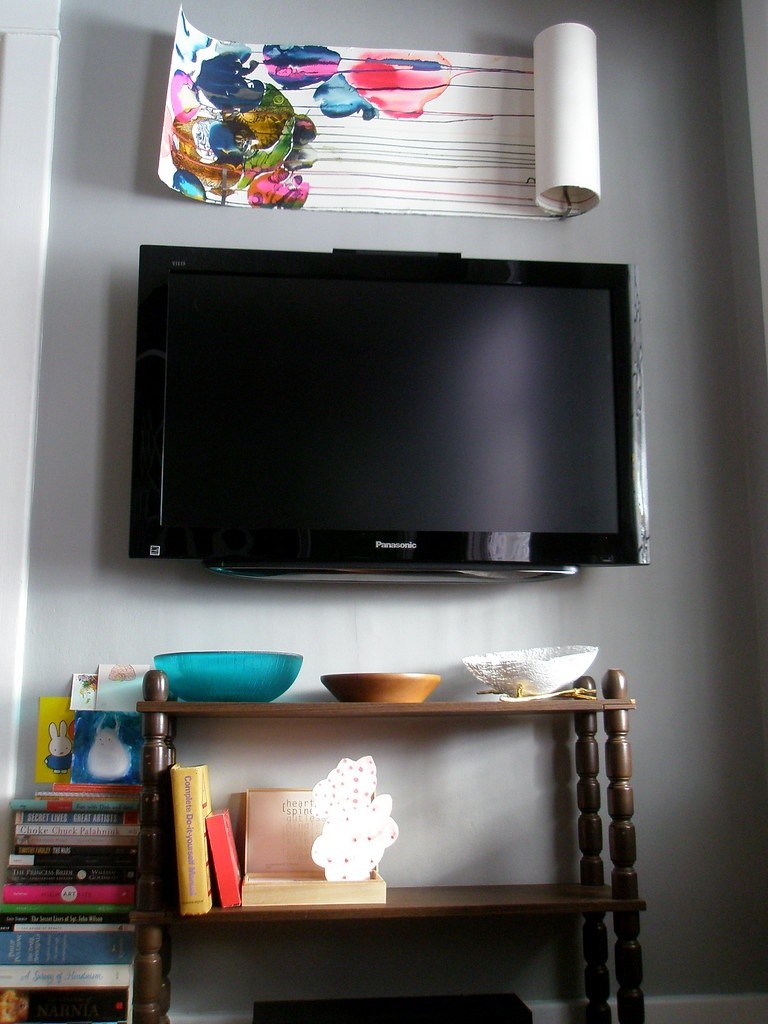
[207,808,242,909]
[240,789,387,907]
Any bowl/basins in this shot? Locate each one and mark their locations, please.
[153,650,304,702]
[319,673,442,703]
[461,646,598,698]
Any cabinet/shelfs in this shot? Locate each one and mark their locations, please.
[134,670,648,1024]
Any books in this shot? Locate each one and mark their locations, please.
[171,761,213,916]
[0,773,144,1023]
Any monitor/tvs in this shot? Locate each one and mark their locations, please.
[128,244,651,583]
